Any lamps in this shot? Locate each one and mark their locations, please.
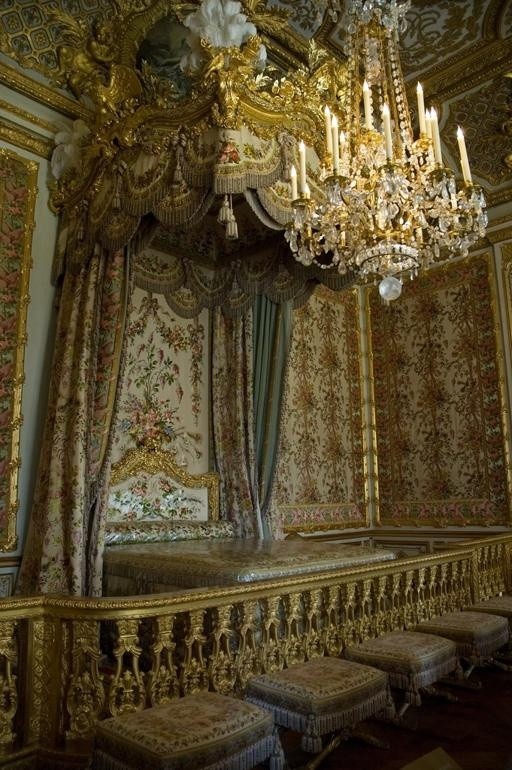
[285,2,489,300]
